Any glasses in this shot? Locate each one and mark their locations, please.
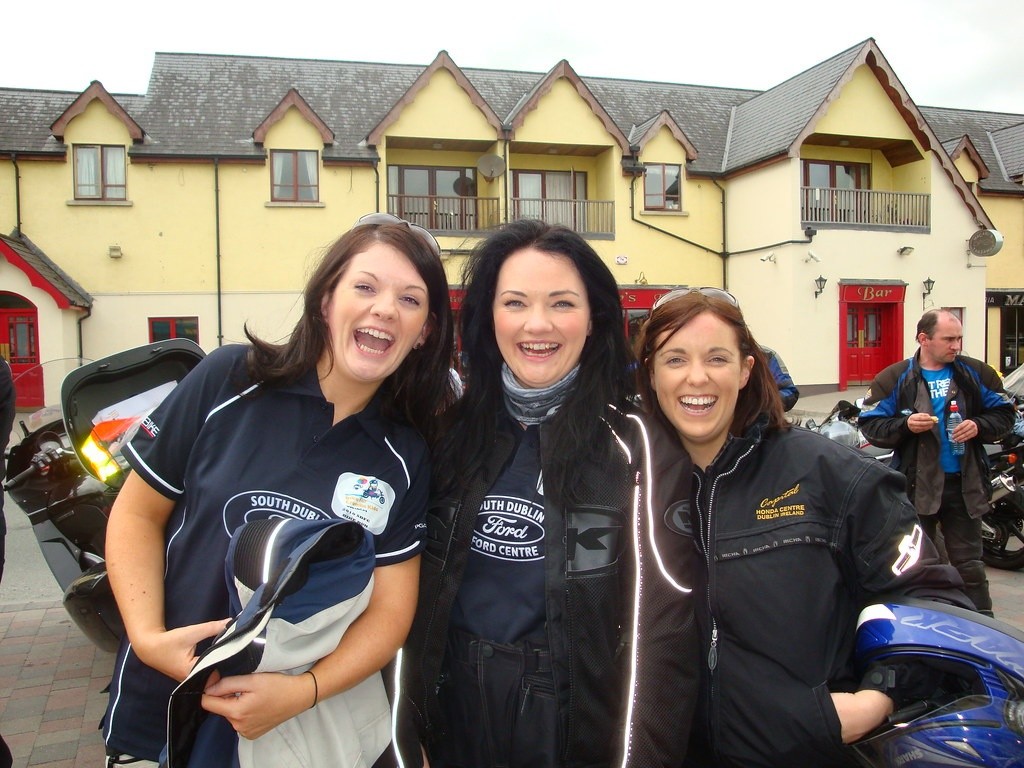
[649,285,742,311]
[353,211,441,257]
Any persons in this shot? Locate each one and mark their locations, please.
[0,355,15,766]
[760,345,801,413]
[100,213,455,767]
[374,219,695,768]
[857,309,1016,617]
[636,291,974,768]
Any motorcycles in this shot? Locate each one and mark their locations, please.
[3,338,207,655]
[805,360,1024,571]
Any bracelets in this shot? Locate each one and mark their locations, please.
[303,671,318,709]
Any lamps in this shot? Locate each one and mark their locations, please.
[815,275,827,298]
[923,278,935,299]
[897,247,914,255]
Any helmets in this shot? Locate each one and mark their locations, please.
[844,595,1024,768]
[817,421,860,448]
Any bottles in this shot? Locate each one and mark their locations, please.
[947,400,965,456]
